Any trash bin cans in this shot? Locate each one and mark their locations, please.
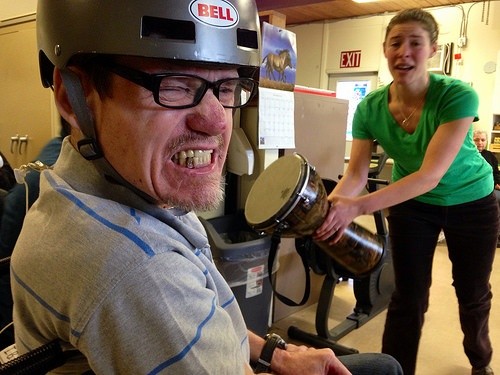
[205,212,272,340]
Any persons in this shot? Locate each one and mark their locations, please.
[472,129,500,191]
[8,0,407,375]
[302,8,500,373]
[0,115,72,352]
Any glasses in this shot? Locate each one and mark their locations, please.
[87,56,259,108]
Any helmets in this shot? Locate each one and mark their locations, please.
[37,0,262,88]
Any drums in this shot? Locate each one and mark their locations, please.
[243,151,388,277]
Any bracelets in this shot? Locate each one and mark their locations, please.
[256,333,288,373]
